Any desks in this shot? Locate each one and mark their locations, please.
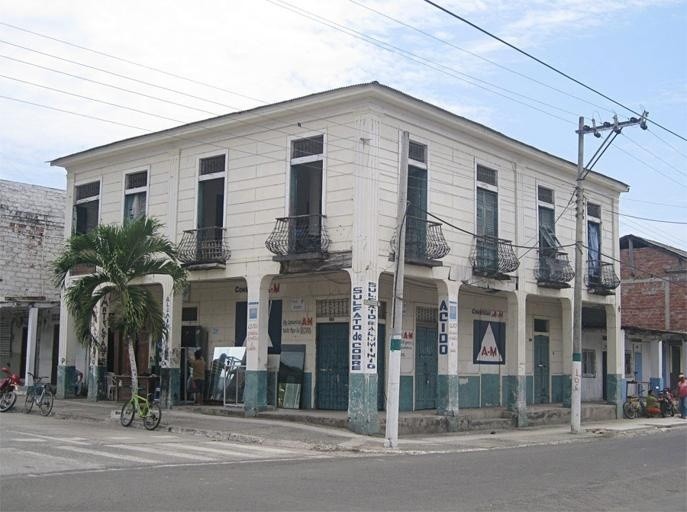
[115,375,154,404]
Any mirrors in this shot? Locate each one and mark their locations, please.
[205,346,247,403]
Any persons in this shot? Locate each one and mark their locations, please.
[187,351,207,406]
[677,375,687,419]
[646,390,662,418]
[74,369,83,396]
[209,353,231,398]
[658,388,673,401]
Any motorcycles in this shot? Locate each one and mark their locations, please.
[655,384,677,417]
[0,361,23,413]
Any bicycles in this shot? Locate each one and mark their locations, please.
[622,389,646,418]
[118,385,163,431]
[24,372,53,417]
